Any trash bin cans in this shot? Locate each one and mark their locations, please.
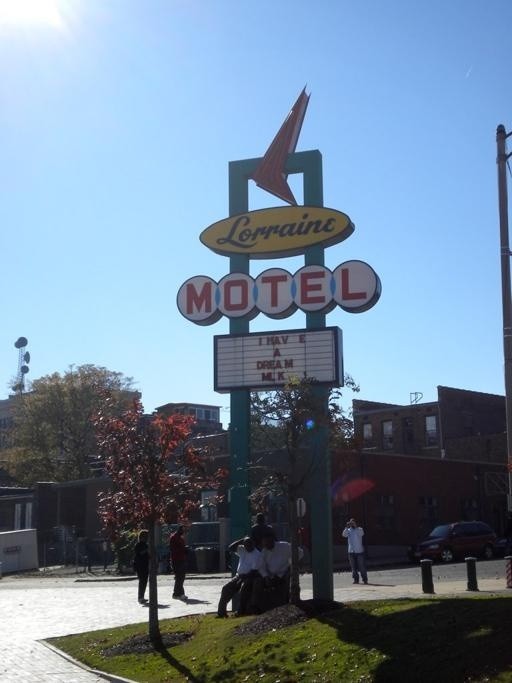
[46,548,57,563]
[194,546,214,572]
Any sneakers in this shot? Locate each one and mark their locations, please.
[353,581,368,584]
[172,595,188,600]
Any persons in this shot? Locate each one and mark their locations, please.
[168,522,189,599]
[250,532,305,614]
[216,535,261,619]
[341,517,369,585]
[133,530,149,604]
[250,512,277,544]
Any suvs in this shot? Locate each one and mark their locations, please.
[408,519,499,565]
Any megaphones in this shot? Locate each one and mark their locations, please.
[21,366,29,373]
[15,337,28,348]
[24,351,30,363]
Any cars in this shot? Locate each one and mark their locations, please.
[497,535,512,557]
